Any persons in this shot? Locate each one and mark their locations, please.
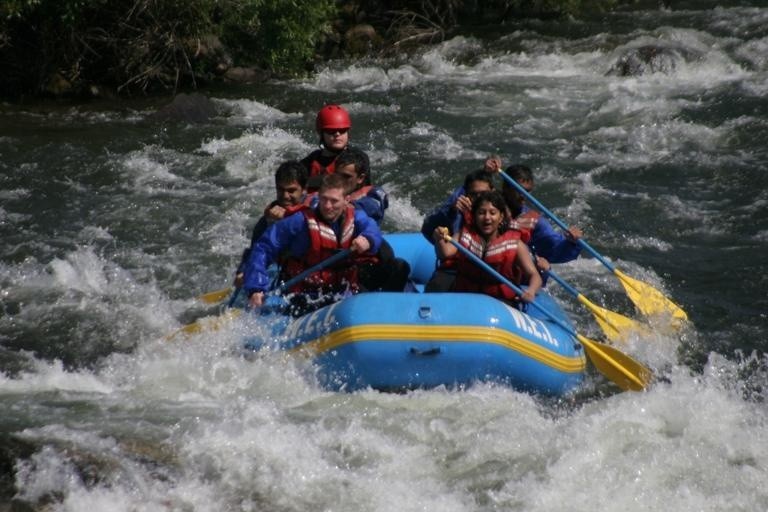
[433,191,543,311]
[439,158,582,288]
[232,161,319,289]
[335,148,411,292]
[298,106,370,194]
[244,173,383,319]
[420,169,551,293]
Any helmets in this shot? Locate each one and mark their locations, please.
[316,103,352,130]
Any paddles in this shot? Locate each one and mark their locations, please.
[441,228,657,392]
[497,168,688,345]
[164,245,356,342]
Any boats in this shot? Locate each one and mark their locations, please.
[232,233,587,393]
